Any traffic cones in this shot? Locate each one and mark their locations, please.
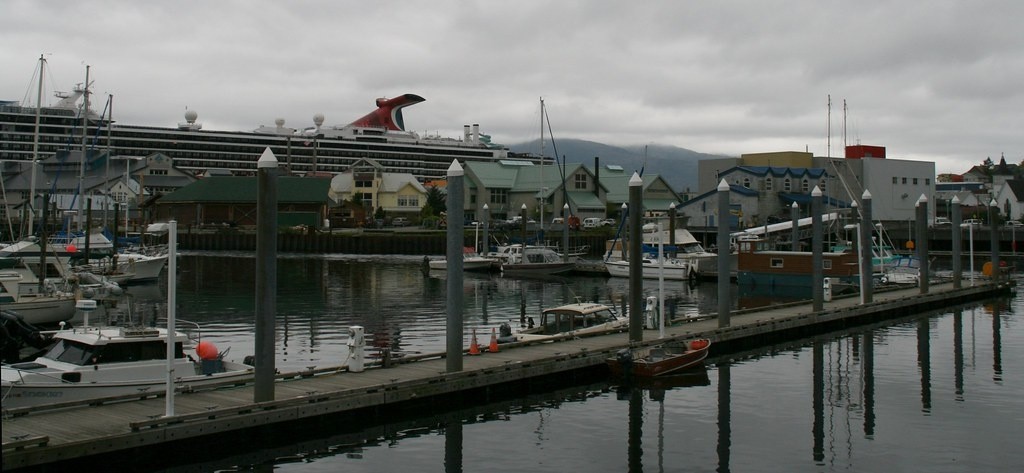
[484,327,501,352]
[468,328,483,356]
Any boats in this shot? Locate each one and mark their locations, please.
[606,336,712,382]
[513,300,631,346]
[486,240,577,277]
[427,254,495,270]
[0,315,258,415]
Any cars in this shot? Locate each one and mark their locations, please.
[391,216,413,227]
[491,216,522,230]
[553,218,563,223]
[929,217,952,228]
[1003,220,1024,227]
[960,218,984,228]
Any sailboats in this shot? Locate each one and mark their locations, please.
[1,49,184,334]
[602,222,708,280]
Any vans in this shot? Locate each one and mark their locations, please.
[582,218,616,229]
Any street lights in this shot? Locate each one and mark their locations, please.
[959,223,977,288]
[1006,220,1017,253]
[843,221,866,307]
[472,222,479,253]
[643,223,667,337]
[144,215,177,417]
[875,222,885,275]
